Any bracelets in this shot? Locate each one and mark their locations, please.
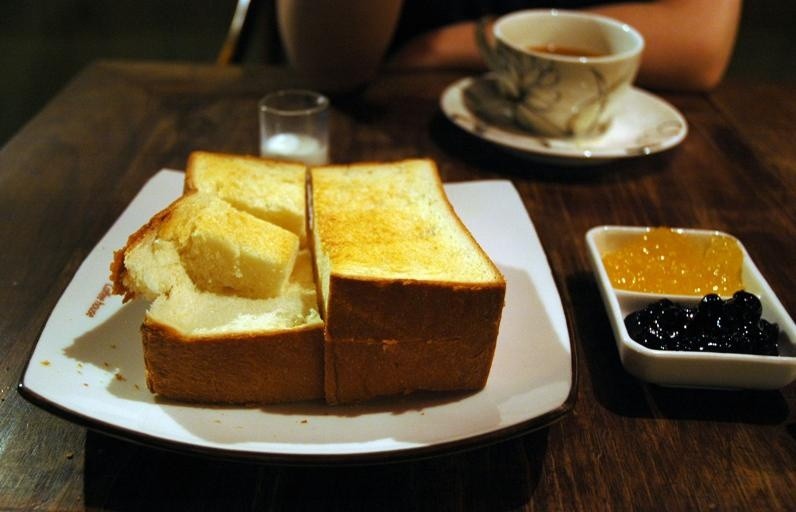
[475,14,502,72]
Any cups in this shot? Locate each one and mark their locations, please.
[256,89,331,168]
[489,8,645,138]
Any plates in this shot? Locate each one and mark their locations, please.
[19,163,574,457]
[439,74,689,161]
[581,218,796,389]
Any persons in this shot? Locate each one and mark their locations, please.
[275,0,743,95]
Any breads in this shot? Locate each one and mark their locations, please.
[307,160,504,410]
[110,152,324,407]
